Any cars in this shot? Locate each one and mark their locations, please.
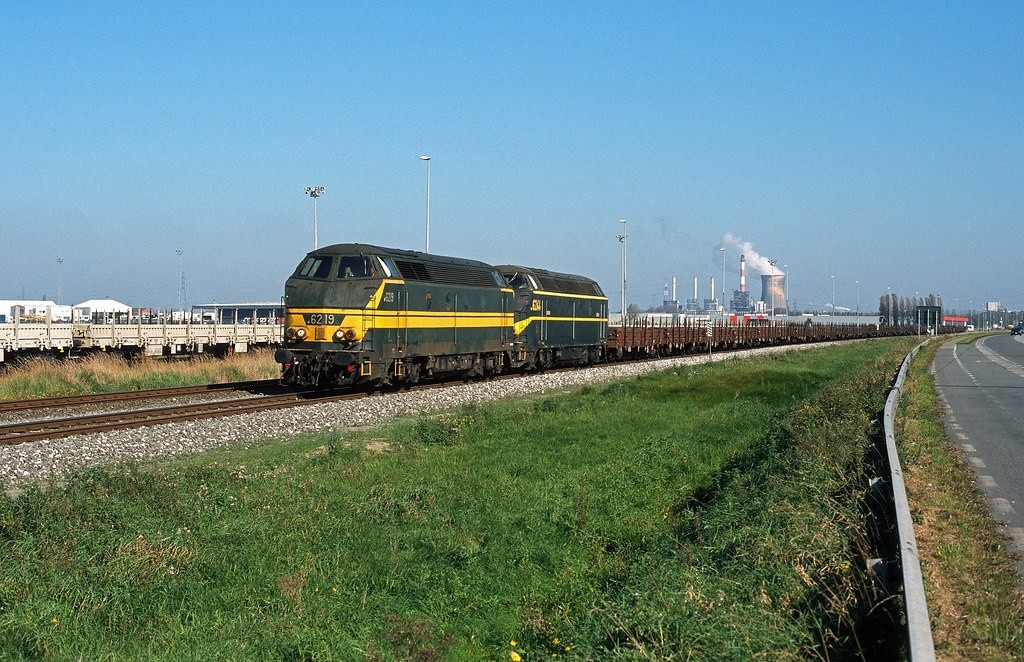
[203,315,215,324]
[1011,328,1022,336]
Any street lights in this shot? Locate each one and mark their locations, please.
[831,275,835,326]
[57,258,64,304]
[619,220,627,317]
[304,186,326,250]
[856,281,860,334]
[616,235,630,315]
[888,287,891,327]
[420,156,430,253]
[720,248,725,306]
[914,291,919,327]
[982,304,984,331]
[784,264,789,322]
[937,295,940,306]
[176,250,184,308]
[280,295,288,345]
[970,301,972,325]
[768,259,778,320]
[955,299,959,327]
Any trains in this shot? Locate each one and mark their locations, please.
[274,243,967,395]
